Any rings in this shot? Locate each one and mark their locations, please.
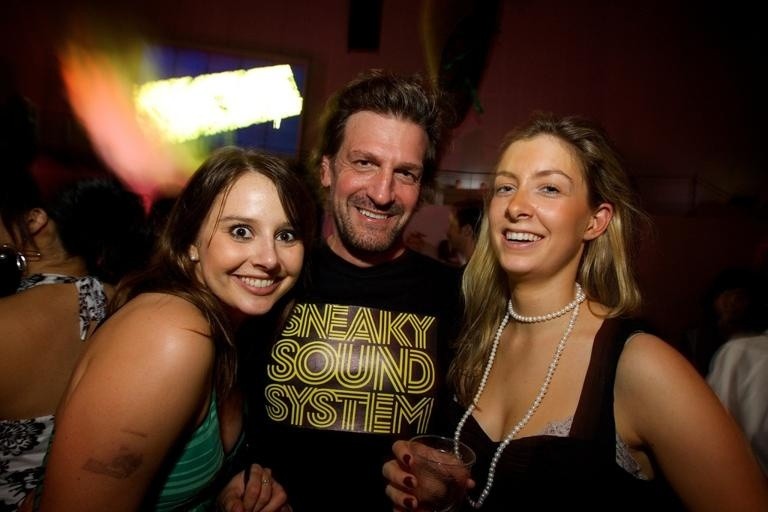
[260,477,273,486]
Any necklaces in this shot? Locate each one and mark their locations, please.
[453,281,586,507]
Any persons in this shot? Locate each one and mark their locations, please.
[705,329,768,468]
[380,110,768,512]
[239,64,470,512]
[41,146,323,512]
[704,266,758,339]
[0,144,149,512]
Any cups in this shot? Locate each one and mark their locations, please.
[407,435,477,512]
